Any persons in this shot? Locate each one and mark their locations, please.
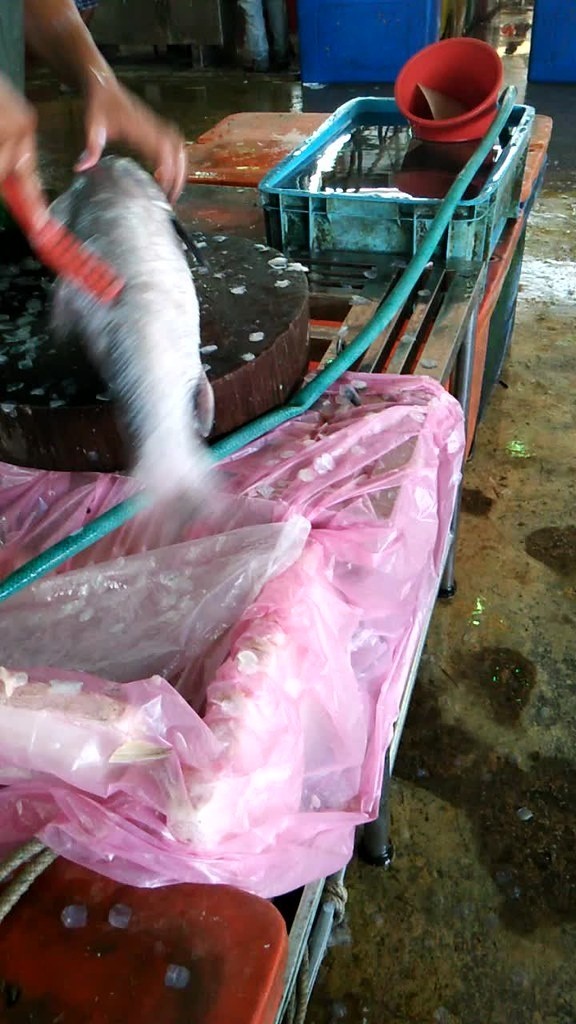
[0,0,187,457]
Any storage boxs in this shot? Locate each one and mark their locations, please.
[527,0,576,85]
[264,87,540,273]
[298,0,441,88]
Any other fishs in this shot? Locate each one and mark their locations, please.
[49,140,231,514]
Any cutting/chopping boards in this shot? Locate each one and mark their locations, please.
[0,230,311,474]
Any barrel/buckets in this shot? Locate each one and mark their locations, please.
[395,38,502,141]
